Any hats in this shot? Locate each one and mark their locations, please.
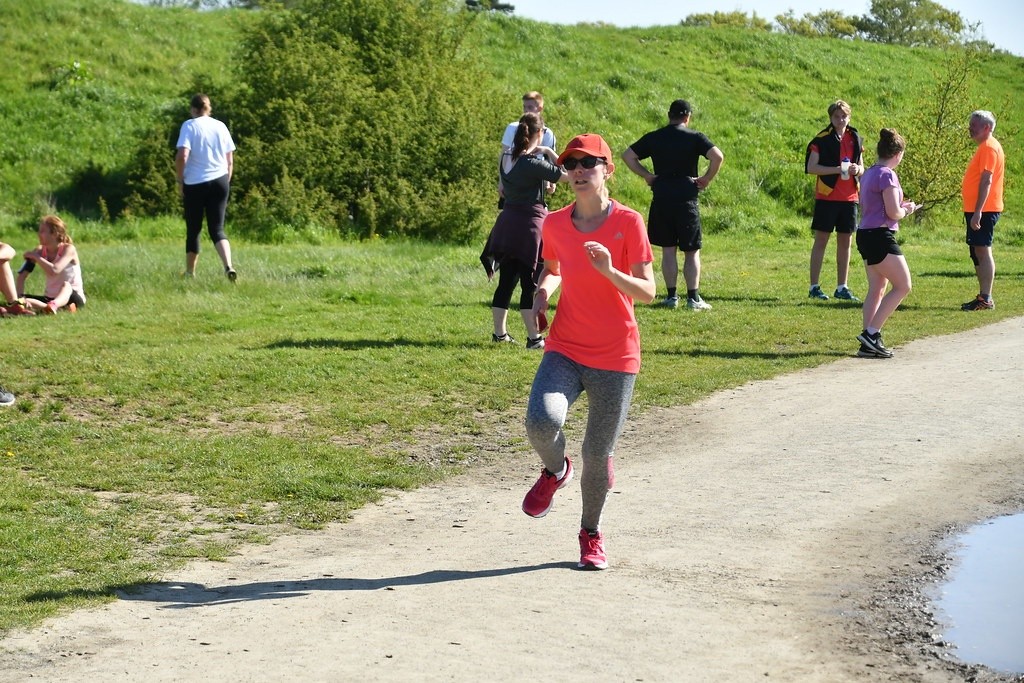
[558,134,613,165]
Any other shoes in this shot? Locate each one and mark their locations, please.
[42,302,56,316]
[66,303,76,312]
[224,267,237,284]
[7,303,36,316]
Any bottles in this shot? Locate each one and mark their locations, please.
[841,157,852,180]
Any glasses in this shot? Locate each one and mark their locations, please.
[561,155,605,171]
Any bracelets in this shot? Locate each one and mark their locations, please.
[853,169,860,176]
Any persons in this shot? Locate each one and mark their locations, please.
[856,127,916,358]
[498,91,557,210]
[0,242,35,316]
[622,99,723,309]
[15,216,86,314]
[521,134,656,569]
[175,93,239,284]
[805,100,864,300]
[481,113,569,349]
[959,110,1004,312]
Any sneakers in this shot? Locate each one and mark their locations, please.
[576,526,608,571]
[963,294,995,311]
[491,332,520,347]
[687,295,712,310]
[809,286,829,300]
[857,345,890,358]
[663,297,678,308]
[521,457,574,519]
[526,337,545,349]
[834,288,859,300]
[855,328,891,355]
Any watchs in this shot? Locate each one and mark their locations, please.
[903,207,909,218]
